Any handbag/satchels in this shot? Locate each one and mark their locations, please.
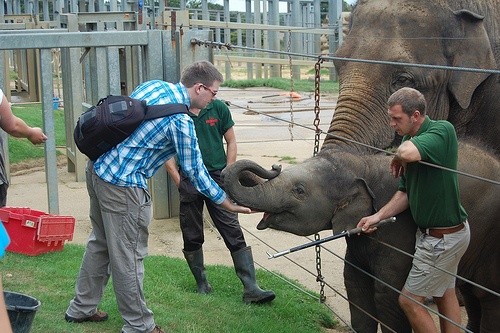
[72,95,190,160]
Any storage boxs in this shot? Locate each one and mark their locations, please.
[0,207,76,256]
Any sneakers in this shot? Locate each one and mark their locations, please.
[149,325,164,333]
[66,307,109,323]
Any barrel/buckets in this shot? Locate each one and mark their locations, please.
[3,290,41,333]
[53,98,59,110]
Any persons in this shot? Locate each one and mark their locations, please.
[357,87,470,333]
[64,61,257,333]
[0,89,48,333]
[164,99,275,303]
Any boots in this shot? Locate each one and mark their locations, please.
[232,245,275,305]
[182,247,216,294]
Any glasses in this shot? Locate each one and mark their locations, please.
[193,82,217,97]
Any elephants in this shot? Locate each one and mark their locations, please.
[219,0,500,333]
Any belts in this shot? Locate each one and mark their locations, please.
[418,222,466,240]
